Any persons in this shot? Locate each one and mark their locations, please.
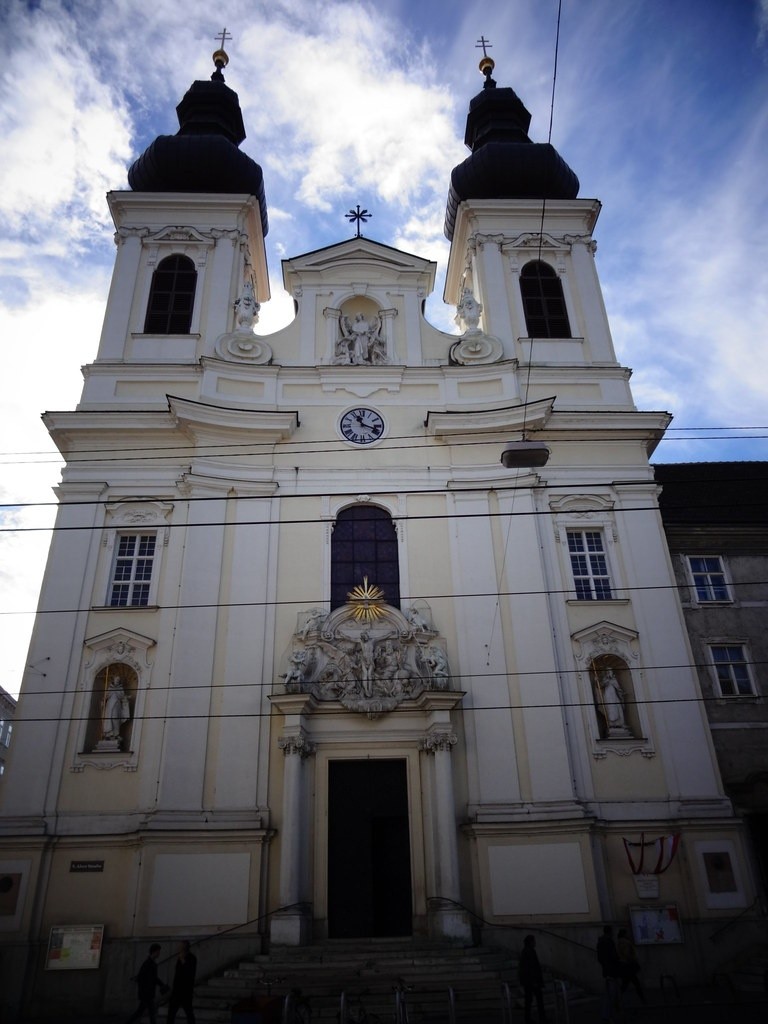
[520,935,547,1024]
[428,646,448,676]
[167,941,197,1024]
[100,675,132,739]
[299,610,319,640]
[126,944,164,1024]
[409,607,430,632]
[284,649,305,685]
[325,630,411,696]
[594,668,624,728]
[353,312,369,360]
[597,925,648,1024]
